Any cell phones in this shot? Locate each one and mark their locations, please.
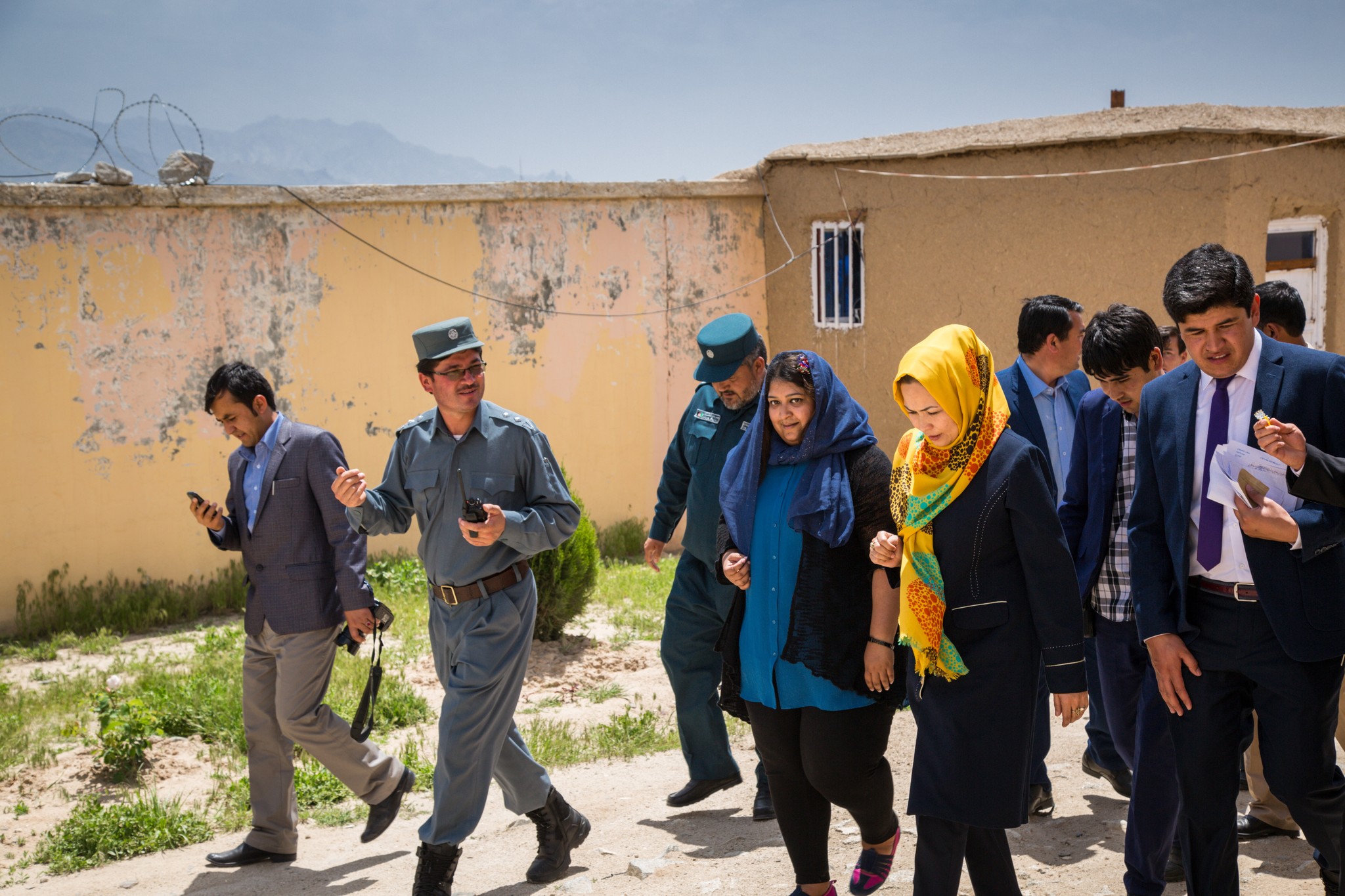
[187,492,220,518]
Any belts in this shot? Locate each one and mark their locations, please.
[430,559,529,605]
[1188,575,1259,602]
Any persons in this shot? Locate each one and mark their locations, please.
[1130,241,1345,896]
[1253,417,1345,510]
[1156,327,1188,371]
[864,329,1088,895]
[1234,279,1345,847]
[1060,303,1192,884]
[711,350,909,896]
[985,293,1134,821]
[327,317,592,896]
[190,363,416,867]
[644,313,782,823]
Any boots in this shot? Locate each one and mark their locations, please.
[413,841,462,896]
[524,784,591,884]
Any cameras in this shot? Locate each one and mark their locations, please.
[333,598,394,655]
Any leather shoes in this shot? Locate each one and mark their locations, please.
[1082,747,1133,798]
[205,841,296,867]
[1027,784,1054,815]
[361,767,415,843]
[1237,815,1300,839]
[1320,868,1340,896]
[667,771,743,807]
[1164,861,1186,882]
[753,788,776,820]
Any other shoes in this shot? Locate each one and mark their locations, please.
[850,826,900,896]
[789,880,838,896]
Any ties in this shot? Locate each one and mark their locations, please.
[1196,373,1237,572]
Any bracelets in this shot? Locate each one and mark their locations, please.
[868,635,895,650]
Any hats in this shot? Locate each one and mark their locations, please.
[412,316,485,361]
[693,313,758,382]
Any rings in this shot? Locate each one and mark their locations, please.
[869,540,878,549]
[365,618,372,627]
[1071,707,1081,716]
[1080,706,1086,711]
[1259,446,1266,452]
